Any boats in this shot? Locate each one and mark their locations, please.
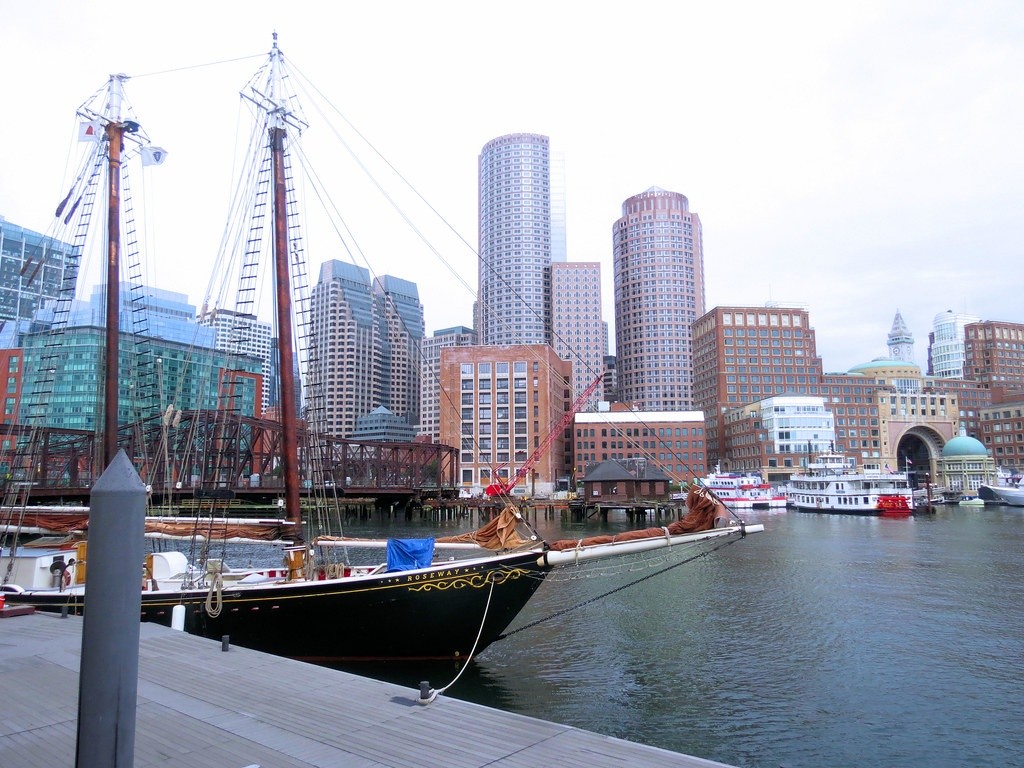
[789,449,916,510]
[699,459,787,508]
[914,483,964,504]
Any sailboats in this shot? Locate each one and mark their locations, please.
[1,68,310,539]
[1,30,765,665]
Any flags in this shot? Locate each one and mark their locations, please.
[140,146,167,168]
[77,121,101,141]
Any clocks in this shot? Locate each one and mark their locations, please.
[908,348,910,353]
[893,347,900,354]
